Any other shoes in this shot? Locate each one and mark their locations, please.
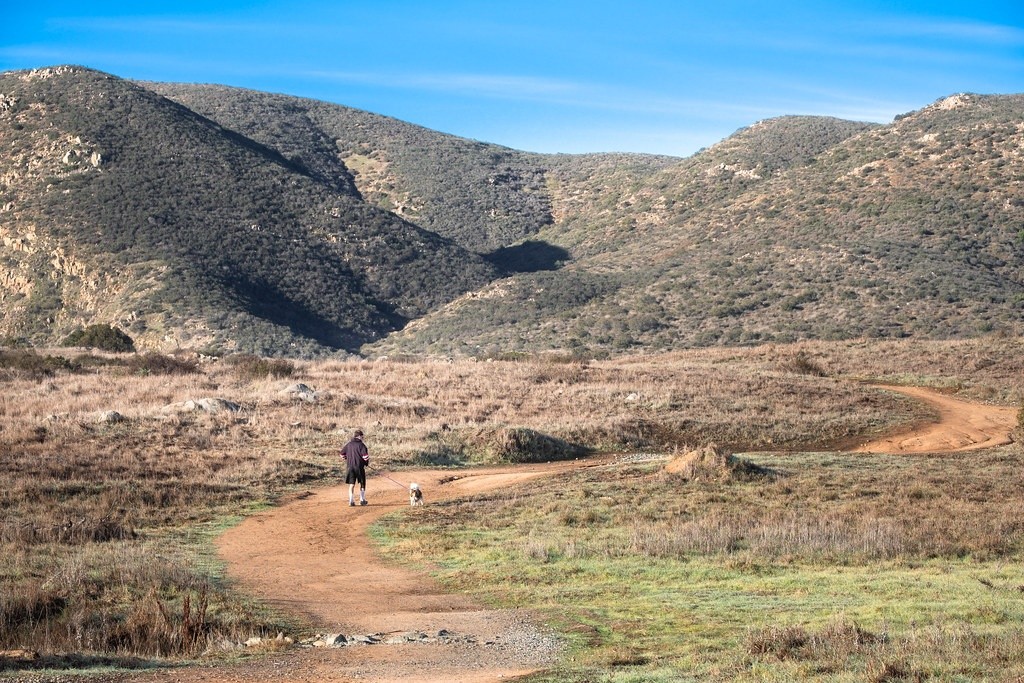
[349,502,355,506]
[360,499,368,506]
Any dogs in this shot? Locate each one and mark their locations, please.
[410,482,424,507]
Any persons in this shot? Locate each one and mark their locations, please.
[340,430,369,506]
